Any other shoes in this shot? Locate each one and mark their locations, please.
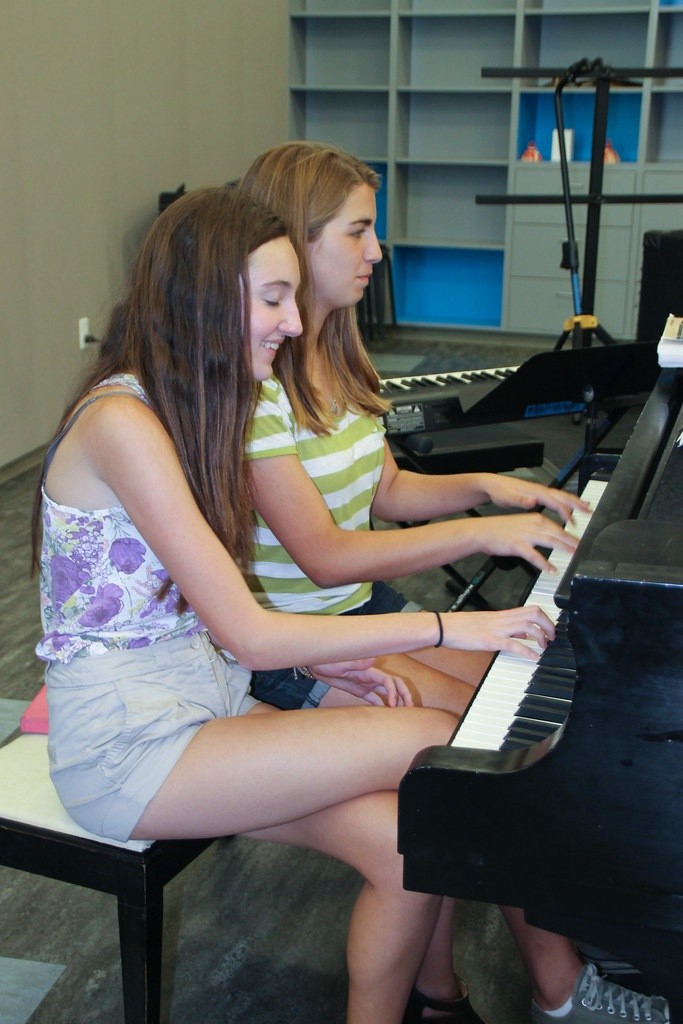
[401,973,485,1024]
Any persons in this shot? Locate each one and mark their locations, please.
[28,189,668,1024]
[242,144,592,1024]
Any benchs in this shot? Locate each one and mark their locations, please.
[0,735,217,1023]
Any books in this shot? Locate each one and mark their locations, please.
[657,315,683,368]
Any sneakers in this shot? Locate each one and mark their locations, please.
[530,962,669,1024]
[576,940,642,973]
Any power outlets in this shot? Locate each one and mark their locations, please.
[78,317,90,350]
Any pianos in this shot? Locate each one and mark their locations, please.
[398,313,683,1024]
[375,362,657,476]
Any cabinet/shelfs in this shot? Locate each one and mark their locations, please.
[287,0,683,349]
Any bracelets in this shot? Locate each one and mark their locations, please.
[433,610,443,648]
[293,667,317,681]
